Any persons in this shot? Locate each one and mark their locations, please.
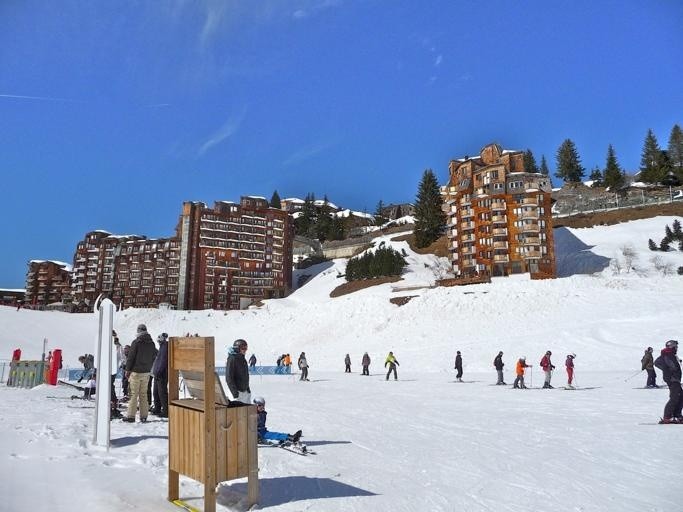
[360,352,371,376]
[77,324,310,447]
[564,352,579,389]
[342,353,353,374]
[654,340,683,424]
[641,346,659,388]
[512,356,533,390]
[492,351,507,386]
[384,352,401,381]
[453,350,466,383]
[539,350,556,389]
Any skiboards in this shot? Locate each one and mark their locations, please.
[638,415,682,424]
[633,383,668,388]
[257,438,315,455]
[510,384,541,389]
[559,385,601,390]
[45,380,85,400]
[537,385,564,389]
[489,381,514,386]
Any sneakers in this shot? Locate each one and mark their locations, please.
[456,378,683,422]
[289,430,302,442]
[110,396,168,422]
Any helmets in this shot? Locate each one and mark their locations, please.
[254,397,265,407]
[457,351,576,360]
[666,340,677,354]
[648,347,653,353]
[234,340,247,353]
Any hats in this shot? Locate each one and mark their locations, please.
[157,333,168,343]
[137,324,147,334]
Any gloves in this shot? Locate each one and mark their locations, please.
[125,371,130,379]
